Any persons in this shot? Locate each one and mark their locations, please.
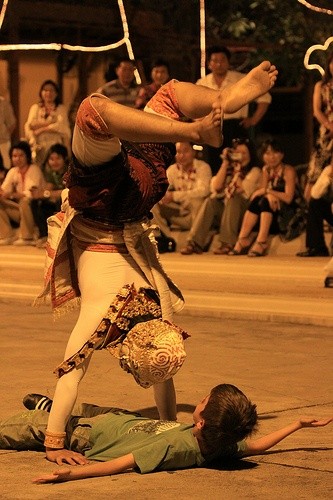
[0,45,333,288]
[31,59,278,466]
[0,383,333,485]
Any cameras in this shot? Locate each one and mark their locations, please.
[228,152,243,162]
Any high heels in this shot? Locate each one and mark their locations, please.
[228,237,267,256]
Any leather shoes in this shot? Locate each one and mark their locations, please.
[295,247,329,257]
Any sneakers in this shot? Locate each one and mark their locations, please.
[0,237,59,248]
[22,394,52,412]
[180,242,230,255]
